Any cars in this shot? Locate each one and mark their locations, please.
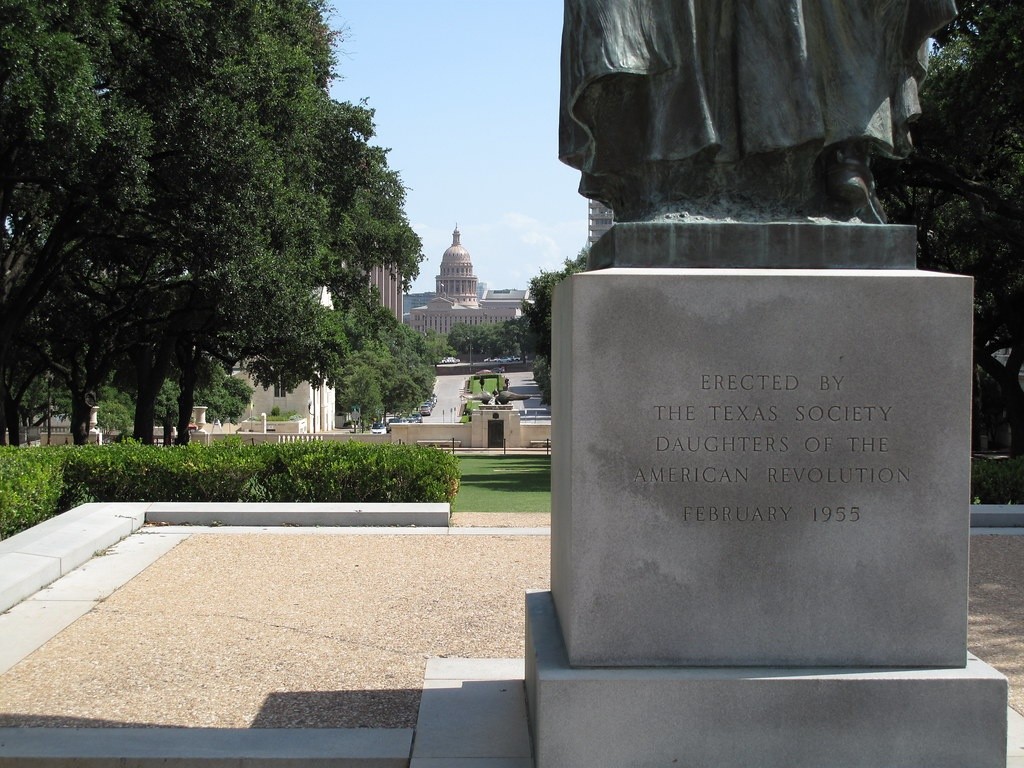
[400,418,410,424]
[484,354,523,363]
[387,418,403,432]
[425,393,439,409]
[406,416,419,424]
[371,423,386,434]
[438,356,460,365]
[420,405,430,416]
[412,413,423,423]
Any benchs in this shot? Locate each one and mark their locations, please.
[530,441,550,448]
[417,440,461,448]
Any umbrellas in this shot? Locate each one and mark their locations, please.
[188,423,198,434]
